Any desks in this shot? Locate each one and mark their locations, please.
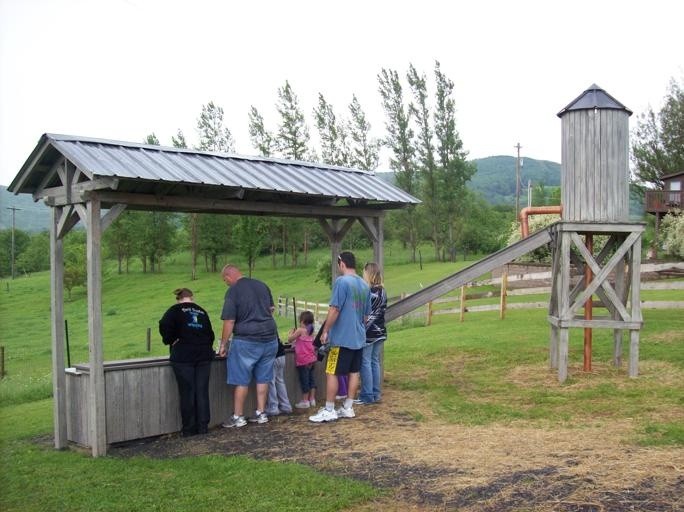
[64,348,328,447]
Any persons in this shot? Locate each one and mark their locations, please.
[264,337,293,416]
[219,264,275,428]
[159,287,216,436]
[288,311,319,409]
[308,252,372,423]
[353,263,387,405]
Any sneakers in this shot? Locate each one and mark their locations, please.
[248,408,269,423]
[294,399,316,409]
[309,407,338,423]
[221,413,247,427]
[336,394,372,417]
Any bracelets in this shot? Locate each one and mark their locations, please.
[220,345,226,347]
